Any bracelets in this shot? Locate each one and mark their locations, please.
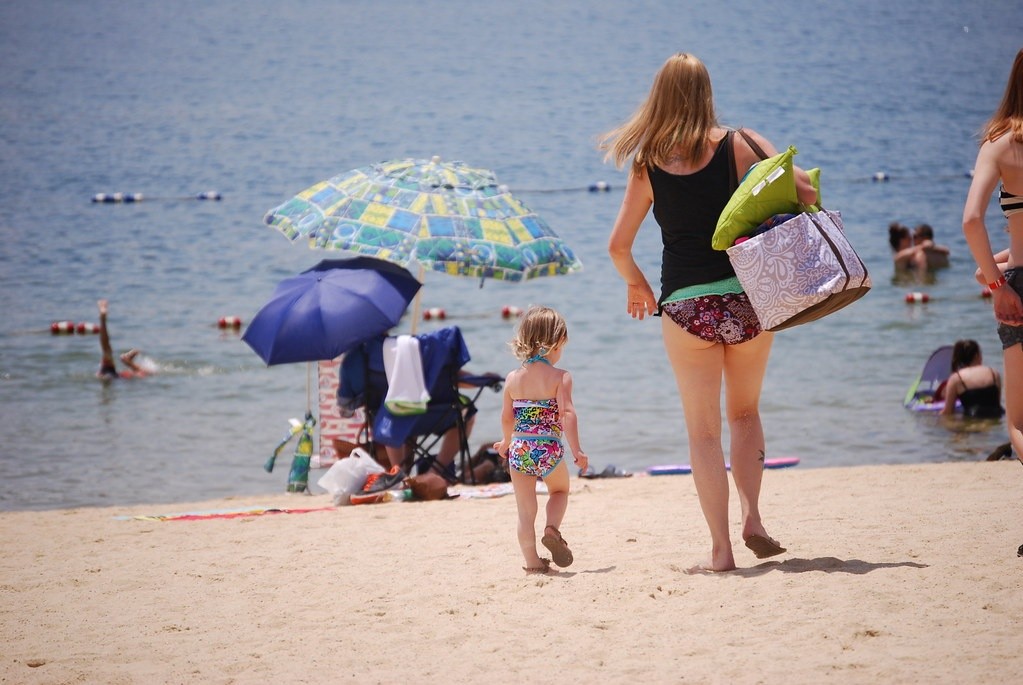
[989,275,1005,290]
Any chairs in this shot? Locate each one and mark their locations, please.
[336,325,507,485]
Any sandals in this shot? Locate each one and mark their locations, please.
[522,557,550,576]
[541,525,574,569]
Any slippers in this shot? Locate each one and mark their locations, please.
[744,534,787,560]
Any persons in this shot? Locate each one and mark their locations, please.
[891,223,950,272]
[939,339,1005,419]
[493,307,589,573]
[97,299,147,376]
[962,48,1023,464]
[600,51,817,573]
[975,249,1010,286]
[373,369,499,477]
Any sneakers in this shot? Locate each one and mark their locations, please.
[350,463,412,506]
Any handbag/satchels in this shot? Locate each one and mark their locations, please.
[726,127,873,332]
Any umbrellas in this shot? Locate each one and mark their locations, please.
[264,155,581,338]
[241,256,424,367]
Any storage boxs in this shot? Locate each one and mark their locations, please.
[416,454,457,485]
